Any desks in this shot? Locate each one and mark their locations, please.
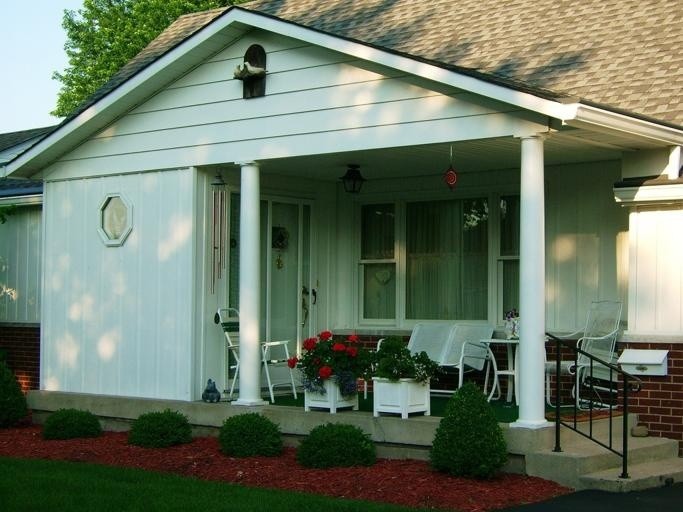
[480,338,552,407]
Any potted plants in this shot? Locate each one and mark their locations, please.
[369,335,449,419]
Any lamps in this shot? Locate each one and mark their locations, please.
[339,164,367,192]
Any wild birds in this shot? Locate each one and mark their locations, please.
[243,62,268,74]
[234,63,242,77]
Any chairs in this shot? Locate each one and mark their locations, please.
[218,307,297,404]
[545,299,624,411]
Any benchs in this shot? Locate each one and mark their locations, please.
[376,322,500,403]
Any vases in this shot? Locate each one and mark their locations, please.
[304,374,359,414]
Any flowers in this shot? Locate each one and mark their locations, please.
[288,328,375,402]
